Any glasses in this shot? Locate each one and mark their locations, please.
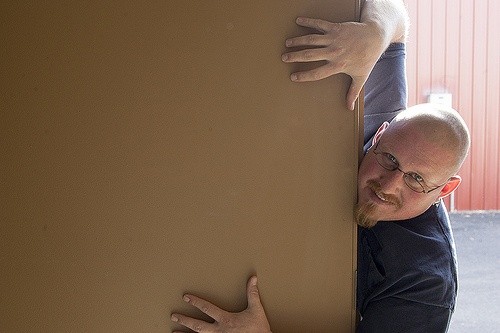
[372,132,451,194]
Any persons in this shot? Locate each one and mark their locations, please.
[167,0,471,333]
